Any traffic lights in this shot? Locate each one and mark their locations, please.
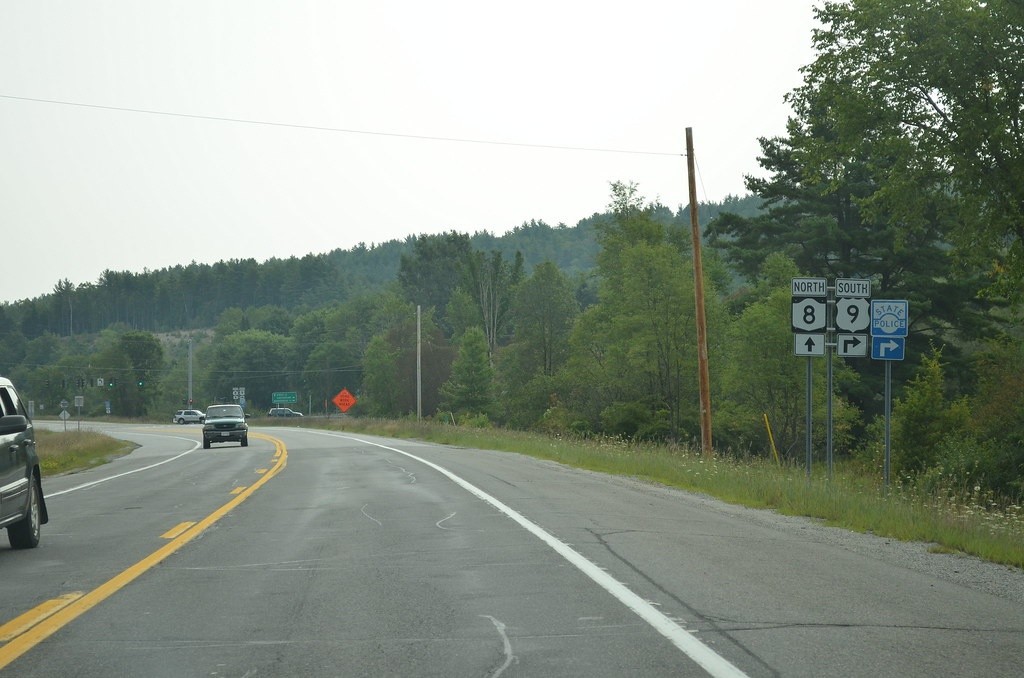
[138,381,144,391]
[81,379,85,387]
[109,384,112,387]
[114,378,119,385]
[46,380,49,389]
[76,378,81,387]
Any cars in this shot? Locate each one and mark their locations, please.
[198,404,250,449]
[268,408,304,417]
[0,377,48,549]
[173,410,206,425]
